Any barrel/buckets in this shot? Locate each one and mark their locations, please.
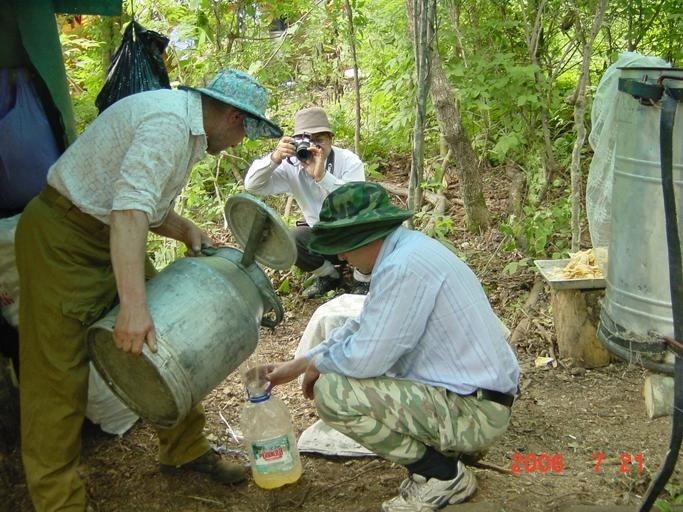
[83,192,300,430]
[242,376,304,489]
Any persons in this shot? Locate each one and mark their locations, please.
[15,67,284,512]
[244,182,520,512]
[244,107,370,303]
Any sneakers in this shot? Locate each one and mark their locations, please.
[302,272,343,300]
[382,459,478,511]
[159,448,247,485]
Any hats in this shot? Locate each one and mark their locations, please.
[307,182,415,255]
[177,68,282,140]
[292,107,334,138]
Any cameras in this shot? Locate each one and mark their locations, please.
[290,139,313,162]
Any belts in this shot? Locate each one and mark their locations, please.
[471,387,515,407]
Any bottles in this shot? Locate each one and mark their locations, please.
[239,389,303,490]
